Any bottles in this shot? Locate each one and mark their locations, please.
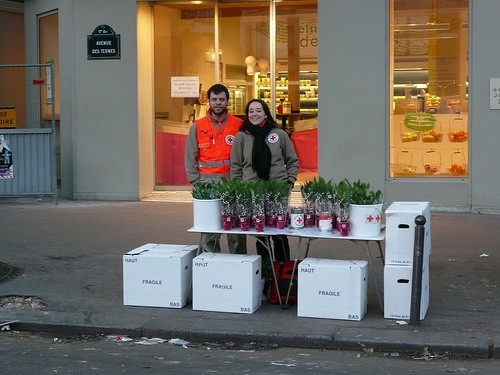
[291,208,305,229]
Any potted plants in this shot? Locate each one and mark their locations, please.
[188,176,385,236]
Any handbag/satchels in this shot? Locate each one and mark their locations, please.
[263,259,302,306]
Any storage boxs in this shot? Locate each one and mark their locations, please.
[121,242,199,309]
[297,257,368,322]
[191,252,263,314]
[383,200,432,319]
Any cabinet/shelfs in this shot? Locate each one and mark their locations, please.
[251,70,318,122]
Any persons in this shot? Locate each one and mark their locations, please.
[231,99,299,262]
[185,84,245,255]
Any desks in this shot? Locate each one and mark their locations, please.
[187,222,386,315]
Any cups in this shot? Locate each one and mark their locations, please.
[319,219,333,232]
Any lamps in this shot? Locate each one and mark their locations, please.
[245,56,256,75]
